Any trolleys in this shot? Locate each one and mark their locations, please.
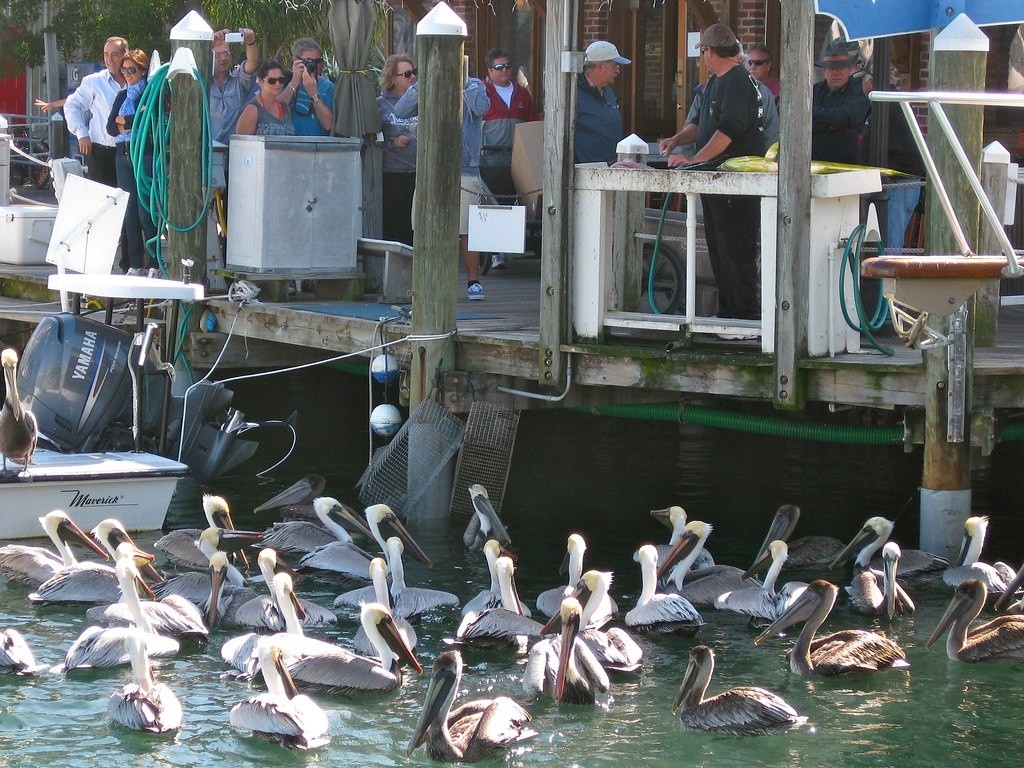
[639,207,716,315]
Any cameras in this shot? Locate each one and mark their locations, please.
[301,58,316,74]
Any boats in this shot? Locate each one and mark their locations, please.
[0,266,204,542]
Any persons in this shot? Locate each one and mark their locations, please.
[659,23,767,339]
[812,37,927,325]
[568,40,622,164]
[235,60,295,136]
[480,47,540,268]
[208,28,259,185]
[106,49,162,279]
[275,37,335,136]
[393,78,490,299]
[683,38,781,154]
[375,52,416,247]
[33,36,129,188]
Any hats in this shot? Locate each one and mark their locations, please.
[813,40,860,69]
[121,49,149,69]
[585,40,631,63]
[694,23,736,49]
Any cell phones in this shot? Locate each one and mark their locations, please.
[225,33,244,43]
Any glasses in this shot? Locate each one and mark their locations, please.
[490,63,512,71]
[396,67,418,78]
[120,66,143,75]
[748,60,767,67]
[215,50,232,58]
[701,46,711,54]
[262,76,285,85]
[297,56,322,65]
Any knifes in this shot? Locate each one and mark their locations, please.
[657,147,668,160]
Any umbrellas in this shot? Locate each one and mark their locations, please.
[330,0,382,157]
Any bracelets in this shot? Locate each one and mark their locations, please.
[311,93,320,102]
[288,83,297,98]
[246,40,256,46]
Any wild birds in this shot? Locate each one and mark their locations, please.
[236,548,337,628]
[109,625,183,732]
[407,651,537,761]
[85,543,208,635]
[336,537,461,623]
[230,643,331,746]
[196,551,259,625]
[251,475,369,540]
[354,558,418,662]
[27,519,164,605]
[251,497,373,554]
[0,509,111,587]
[457,485,1024,739]
[64,558,181,669]
[0,349,38,477]
[152,526,265,603]
[153,493,249,570]
[0,629,37,675]
[298,504,434,579]
[221,575,423,696]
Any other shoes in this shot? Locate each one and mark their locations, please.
[716,333,758,340]
[125,267,148,276]
[148,266,169,280]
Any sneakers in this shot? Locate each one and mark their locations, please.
[491,253,507,268]
[468,282,485,299]
